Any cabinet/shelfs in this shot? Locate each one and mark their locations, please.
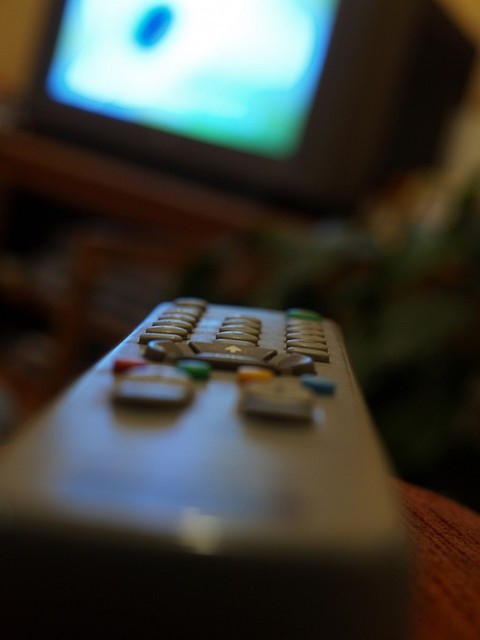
[0,130,369,399]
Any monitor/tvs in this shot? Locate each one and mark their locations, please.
[24,0,476,215]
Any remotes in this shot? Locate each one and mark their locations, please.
[2,295,415,637]
